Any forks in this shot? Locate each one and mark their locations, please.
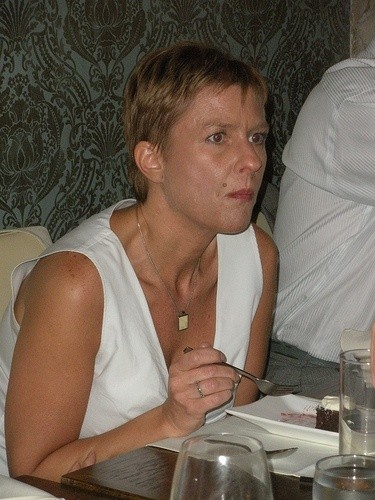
[183,347,298,397]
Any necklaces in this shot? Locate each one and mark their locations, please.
[136,207,200,331]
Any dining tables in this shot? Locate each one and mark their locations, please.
[61,389,375,500]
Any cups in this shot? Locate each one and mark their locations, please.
[168,432,273,500]
[336,347,375,458]
[311,453,375,500]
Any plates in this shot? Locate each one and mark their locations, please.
[225,393,375,451]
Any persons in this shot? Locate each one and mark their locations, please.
[263,37,375,394]
[0,41,281,482]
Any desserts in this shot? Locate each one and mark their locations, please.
[316,396,361,432]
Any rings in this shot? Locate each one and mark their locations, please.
[196,383,203,397]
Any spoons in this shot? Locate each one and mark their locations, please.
[204,437,298,460]
